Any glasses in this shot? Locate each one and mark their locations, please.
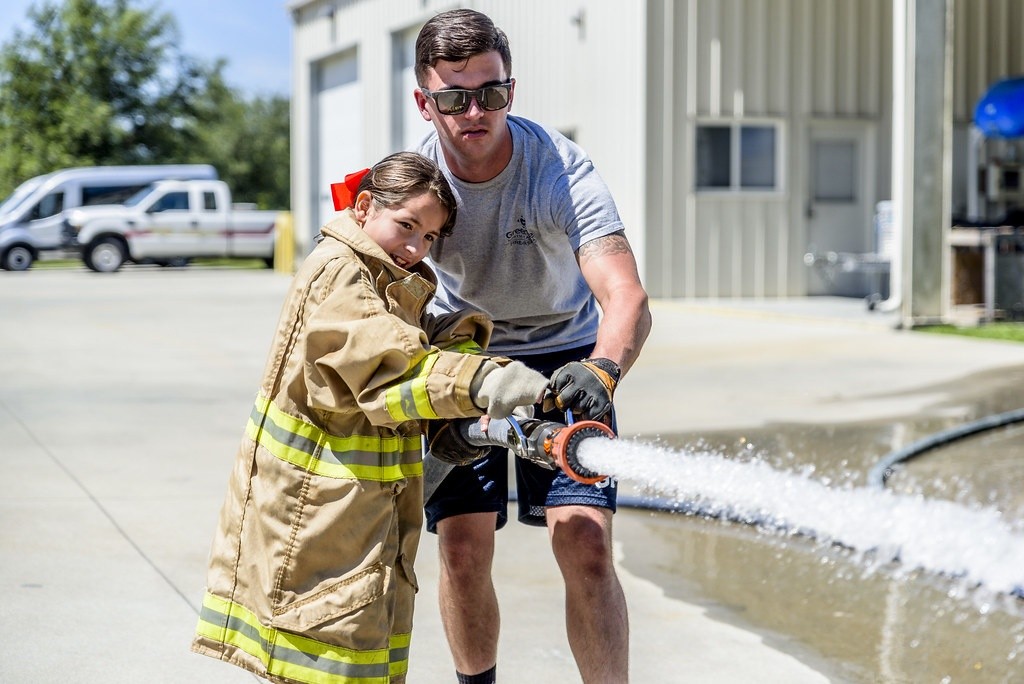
[420,78,512,115]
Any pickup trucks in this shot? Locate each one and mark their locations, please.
[59,181,285,273]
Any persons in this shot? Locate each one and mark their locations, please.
[192,152,551,684]
[405,10,652,683]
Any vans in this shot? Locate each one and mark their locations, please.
[0,165,218,270]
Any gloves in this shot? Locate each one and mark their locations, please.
[549,357,621,423]
[423,417,492,466]
